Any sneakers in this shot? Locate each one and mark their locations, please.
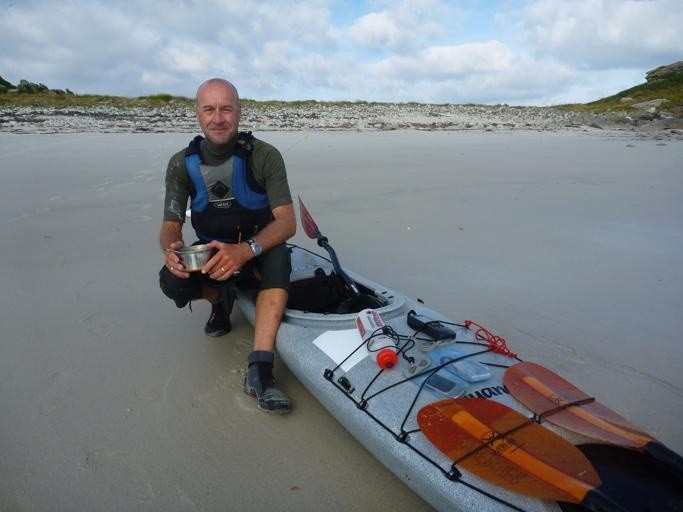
[204,288,236,338]
[243,366,292,415]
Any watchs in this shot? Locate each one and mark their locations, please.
[245,237,263,258]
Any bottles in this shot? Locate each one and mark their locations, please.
[354,308,398,371]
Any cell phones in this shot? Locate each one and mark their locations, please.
[425,374,467,399]
[442,356,492,383]
[407,315,456,341]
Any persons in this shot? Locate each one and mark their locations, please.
[157,78,296,414]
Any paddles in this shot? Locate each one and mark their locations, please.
[298,196,366,313]
[503,362,683,472]
[417,397,628,511]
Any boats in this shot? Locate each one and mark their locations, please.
[229,237,683,512]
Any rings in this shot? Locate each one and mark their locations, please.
[169,266,173,272]
[220,267,226,272]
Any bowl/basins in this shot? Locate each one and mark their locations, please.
[172,242,214,272]
